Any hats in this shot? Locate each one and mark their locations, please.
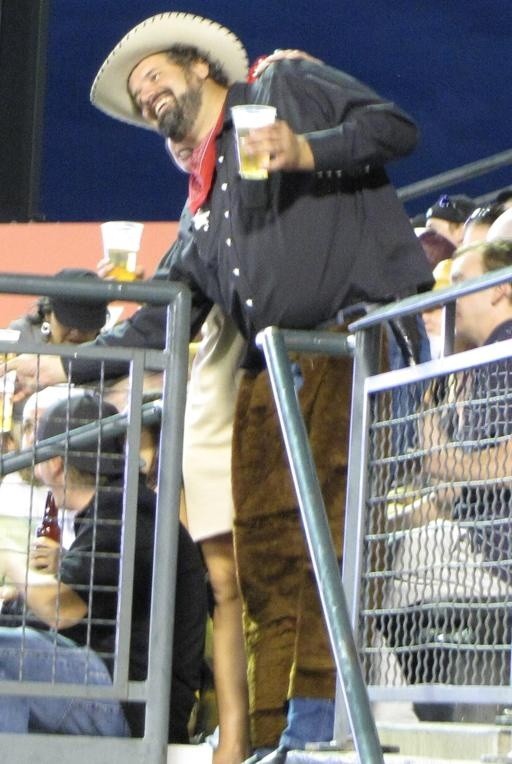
[424,193,479,223]
[34,385,147,478]
[49,265,108,329]
[87,7,250,134]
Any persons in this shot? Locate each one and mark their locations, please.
[0,9,437,763]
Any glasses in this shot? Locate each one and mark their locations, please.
[437,192,452,209]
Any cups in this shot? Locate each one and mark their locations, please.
[228,103,276,180]
[99,219,144,282]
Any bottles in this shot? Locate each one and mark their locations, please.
[34,489,62,570]
